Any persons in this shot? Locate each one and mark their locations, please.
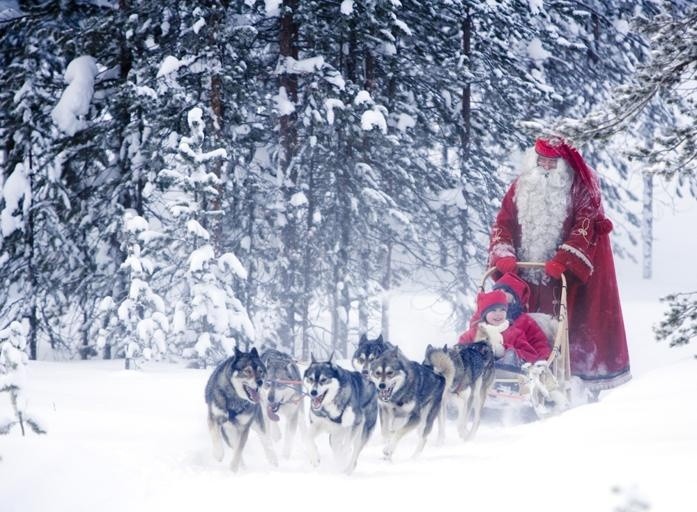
[460,291,538,368]
[469,271,553,362]
[488,131,631,382]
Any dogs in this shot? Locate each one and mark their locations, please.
[204,333,486,473]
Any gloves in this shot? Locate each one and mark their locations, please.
[495,257,516,273]
[544,259,565,280]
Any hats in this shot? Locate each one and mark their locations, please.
[534,135,566,158]
[470,291,507,320]
[495,273,530,309]
